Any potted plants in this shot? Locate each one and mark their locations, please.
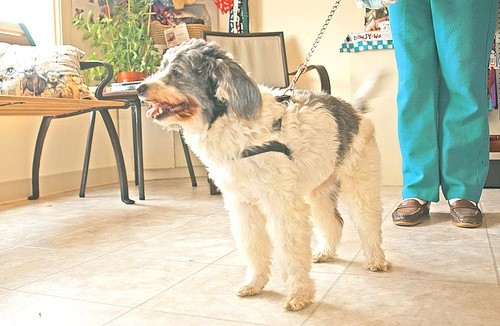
[71,0,164,82]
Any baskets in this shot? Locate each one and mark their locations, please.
[145,21,208,44]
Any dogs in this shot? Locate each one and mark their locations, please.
[135,37,391,312]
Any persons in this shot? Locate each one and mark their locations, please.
[388,0,498,228]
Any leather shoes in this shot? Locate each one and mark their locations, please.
[392,199,431,226]
[448,199,482,227]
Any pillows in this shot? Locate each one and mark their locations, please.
[0,42,99,101]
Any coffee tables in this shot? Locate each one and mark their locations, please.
[79,90,197,200]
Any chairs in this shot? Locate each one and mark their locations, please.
[0,22,137,205]
[201,30,331,196]
